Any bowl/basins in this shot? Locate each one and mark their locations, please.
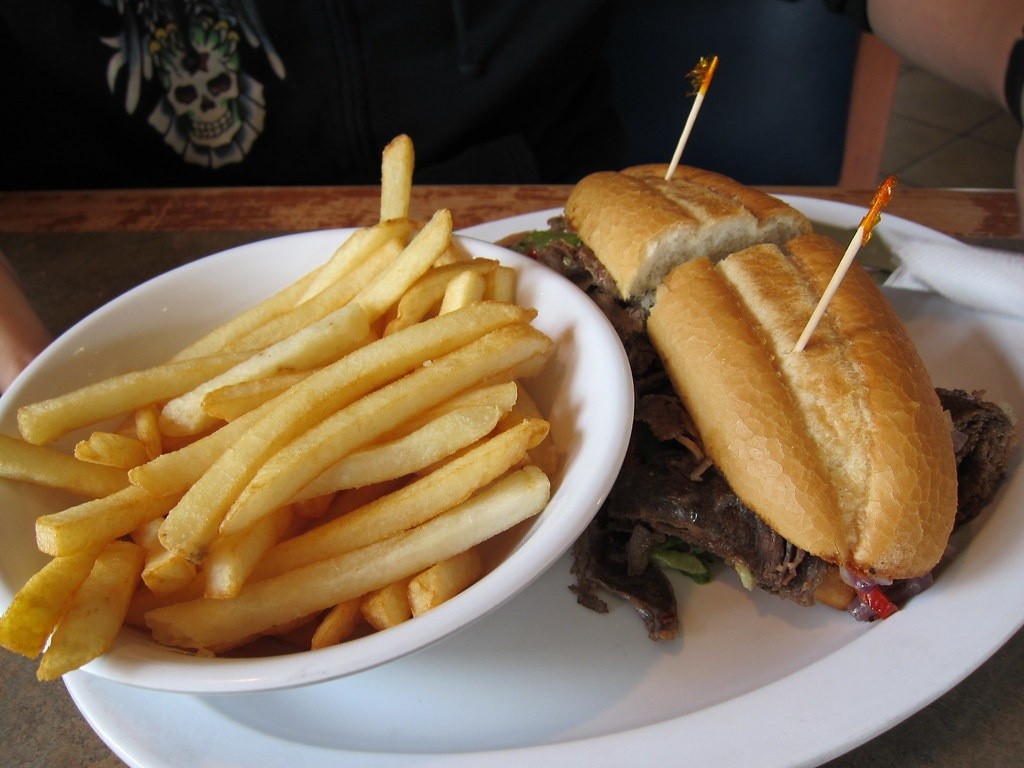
[0,228,634,692]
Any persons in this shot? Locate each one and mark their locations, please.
[0,0,1024,398]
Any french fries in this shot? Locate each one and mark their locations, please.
[1,136,557,682]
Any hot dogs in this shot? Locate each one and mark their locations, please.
[514,159,955,615]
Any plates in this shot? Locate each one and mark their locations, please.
[57,193,1024,768]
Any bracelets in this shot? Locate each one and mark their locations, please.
[1004,37,1024,128]
